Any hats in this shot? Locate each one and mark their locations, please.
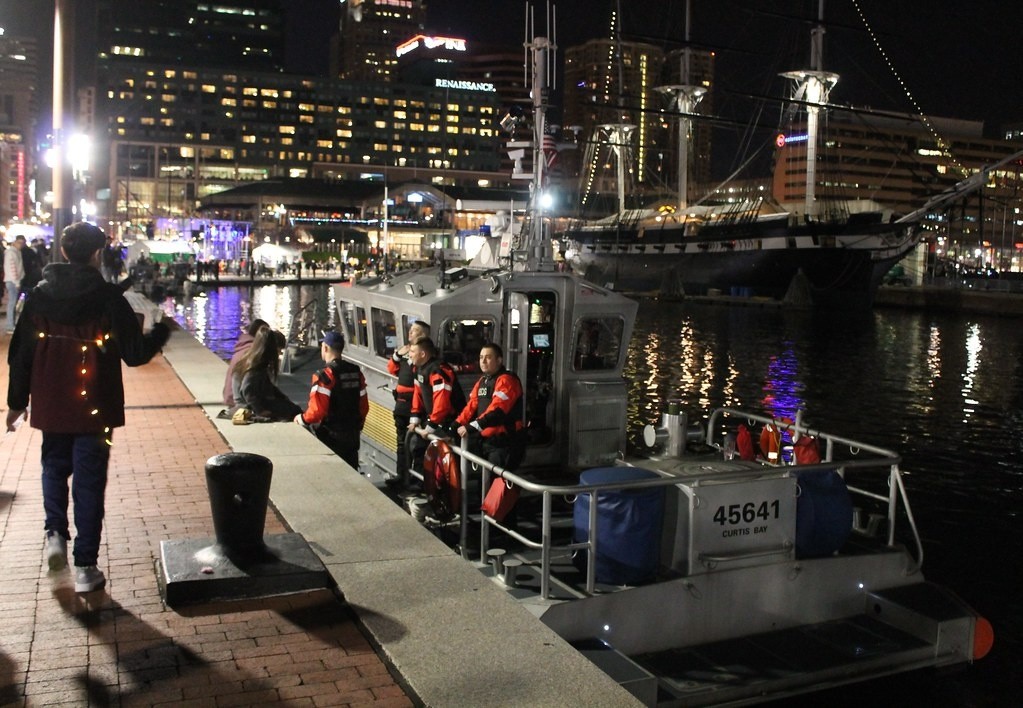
[249,319,269,337]
[319,332,346,348]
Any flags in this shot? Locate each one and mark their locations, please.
[542,118,559,171]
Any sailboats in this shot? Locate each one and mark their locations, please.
[551,0,1023,304]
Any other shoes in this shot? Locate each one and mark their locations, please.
[487,528,503,540]
[424,511,461,524]
[384,475,419,487]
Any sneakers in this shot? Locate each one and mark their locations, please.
[44,530,68,569]
[74,566,106,593]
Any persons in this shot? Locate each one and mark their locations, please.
[449,344,524,549]
[101,236,400,289]
[5,222,179,592]
[407,336,467,469]
[0,235,48,335]
[293,331,369,473]
[383,321,431,487]
[222,319,305,423]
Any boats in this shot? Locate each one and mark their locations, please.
[270,0,995,707]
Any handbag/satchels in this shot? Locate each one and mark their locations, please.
[231,408,254,425]
